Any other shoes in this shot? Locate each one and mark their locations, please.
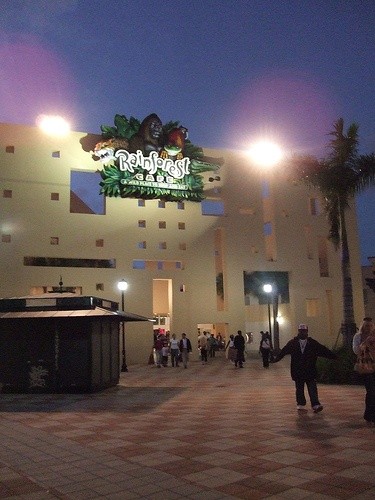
[363,413,375,422]
[296,405,306,410]
[313,406,323,414]
[240,366,243,368]
[235,364,237,367]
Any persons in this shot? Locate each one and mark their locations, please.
[225,330,245,368]
[180,333,192,369]
[276,324,338,412]
[153,329,180,367]
[198,331,222,363]
[352,317,375,423]
[169,333,180,367]
[260,331,273,368]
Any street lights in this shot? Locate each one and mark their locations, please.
[118,279,129,372]
[263,284,272,348]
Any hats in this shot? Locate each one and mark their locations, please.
[298,323,308,329]
